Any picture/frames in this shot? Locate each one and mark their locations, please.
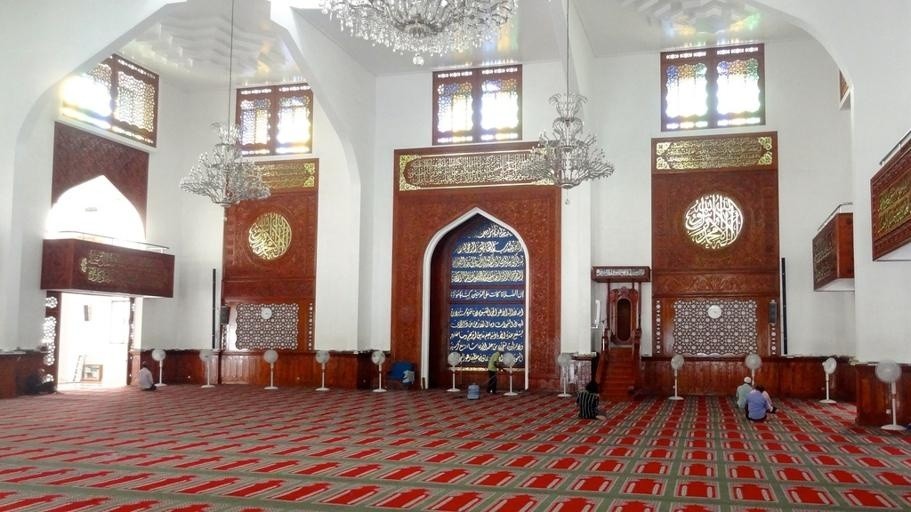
[0,353,44,399]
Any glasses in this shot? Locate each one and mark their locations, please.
[524,0,615,205]
[318,0,517,65]
[180,0,271,222]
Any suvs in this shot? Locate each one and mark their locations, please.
[467,381,480,399]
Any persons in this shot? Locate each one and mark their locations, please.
[736,377,754,409]
[136,361,156,391]
[745,384,769,422]
[575,381,607,420]
[486,347,506,395]
[760,385,779,413]
[24,367,56,394]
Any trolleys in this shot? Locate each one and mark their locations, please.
[503,353,517,395]
[819,358,837,403]
[745,354,761,386]
[668,355,684,400]
[446,352,461,392]
[151,346,280,389]
[557,354,573,397]
[371,351,388,393]
[315,350,330,391]
[875,359,906,431]
